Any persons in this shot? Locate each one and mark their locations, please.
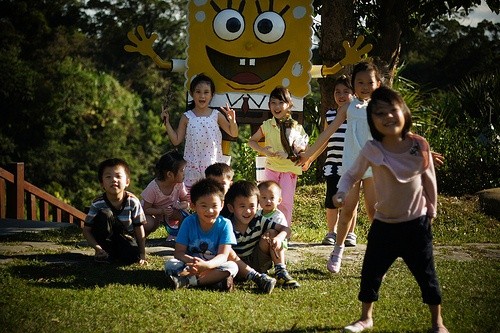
[204,163,236,220]
[82,159,147,265]
[300,75,362,247]
[224,180,301,294]
[333,87,449,333]
[160,73,239,203]
[249,86,308,239]
[138,155,190,243]
[163,178,240,291]
[295,61,445,280]
[255,181,289,252]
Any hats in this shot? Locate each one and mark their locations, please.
[163,207,189,236]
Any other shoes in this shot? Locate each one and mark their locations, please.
[169,274,189,291]
[345,232,358,245]
[327,250,342,273]
[433,325,449,333]
[322,232,336,245]
[95,247,114,264]
[256,273,276,295]
[274,269,299,288]
[345,320,372,333]
